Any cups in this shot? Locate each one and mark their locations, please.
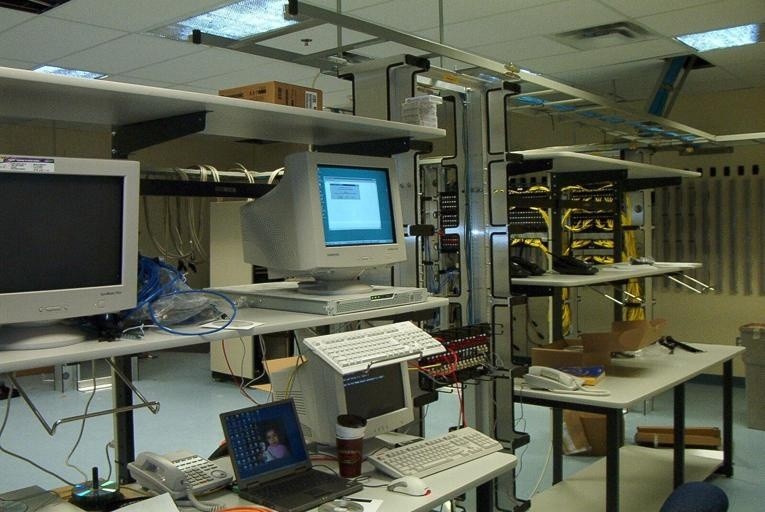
[333,413,366,477]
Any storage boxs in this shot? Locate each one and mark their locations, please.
[561,410,627,455]
[531,318,666,368]
[218,80,324,115]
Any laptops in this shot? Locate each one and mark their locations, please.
[219,398,363,512]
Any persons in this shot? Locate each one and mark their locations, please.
[253,421,291,463]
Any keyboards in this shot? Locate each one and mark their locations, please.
[303,320,447,376]
[368,426,504,479]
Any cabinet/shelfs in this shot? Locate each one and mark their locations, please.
[0,66,448,376]
[505,147,702,287]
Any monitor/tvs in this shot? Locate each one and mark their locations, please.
[0,154,140,351]
[237,151,407,295]
[270,350,415,459]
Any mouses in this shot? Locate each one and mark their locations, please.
[387,475,431,497]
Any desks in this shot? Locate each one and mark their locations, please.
[513,337,745,511]
[39,430,517,510]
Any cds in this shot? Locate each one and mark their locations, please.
[72,478,120,497]
[318,500,364,512]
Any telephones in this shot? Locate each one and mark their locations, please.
[127,451,233,500]
[523,365,585,392]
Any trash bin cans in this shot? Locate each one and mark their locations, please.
[736,323,765,431]
